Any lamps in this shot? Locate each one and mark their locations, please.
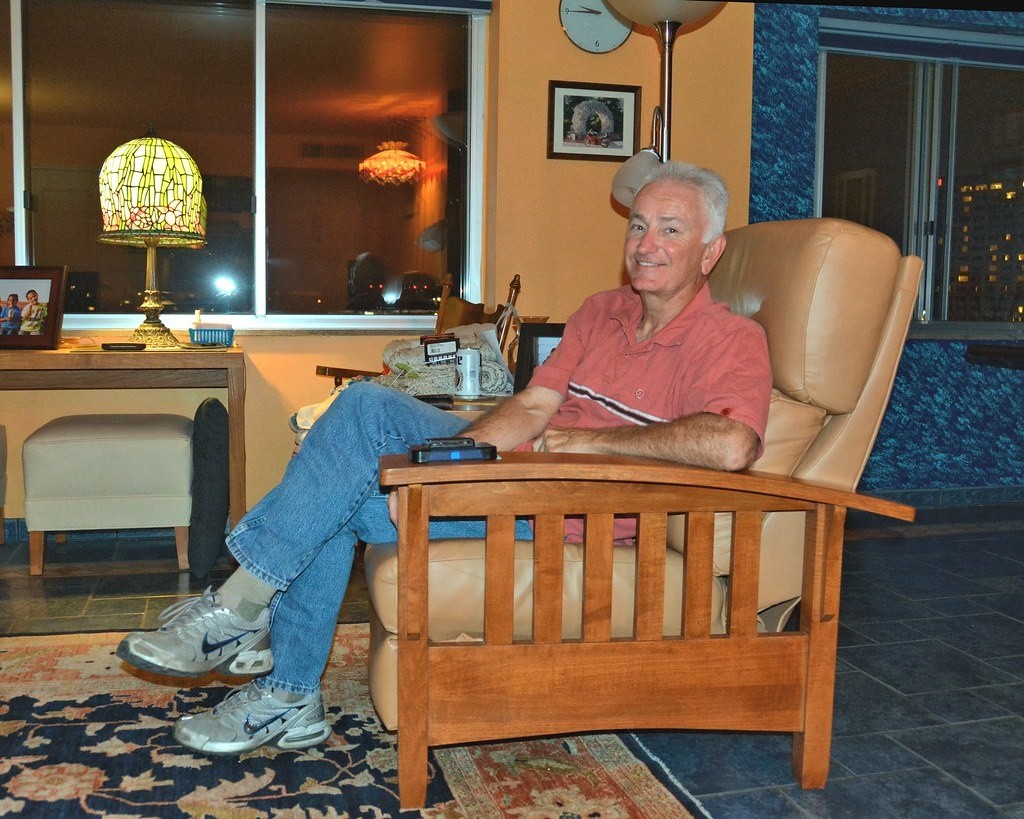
[96,136,203,351]
[357,141,426,188]
[607,0,727,210]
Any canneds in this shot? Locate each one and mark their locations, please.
[455,347,482,398]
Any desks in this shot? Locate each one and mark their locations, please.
[0,342,246,532]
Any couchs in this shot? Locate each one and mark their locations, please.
[366,218,924,811]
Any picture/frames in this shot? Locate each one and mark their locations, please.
[546,79,642,163]
[0,264,69,350]
[513,322,566,395]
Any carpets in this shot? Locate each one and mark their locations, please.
[0,623,714,819]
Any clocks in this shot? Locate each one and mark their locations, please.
[559,0,635,54]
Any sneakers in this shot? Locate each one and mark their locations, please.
[173,680,332,754]
[116,586,274,679]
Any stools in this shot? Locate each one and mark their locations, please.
[22,414,194,573]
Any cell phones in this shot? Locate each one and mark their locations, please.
[102,344,146,350]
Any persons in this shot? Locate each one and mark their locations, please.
[115,161,774,759]
[17,289,48,336]
[0,294,21,336]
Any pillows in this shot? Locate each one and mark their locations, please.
[705,217,901,414]
